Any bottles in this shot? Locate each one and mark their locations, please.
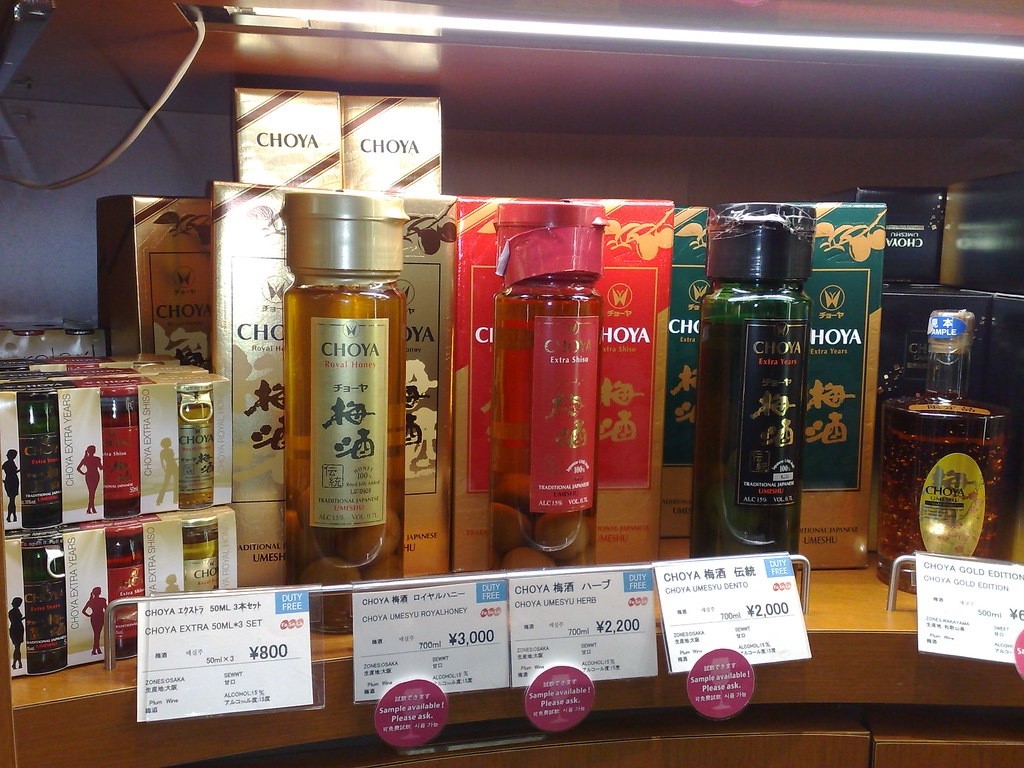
[17,390,61,528]
[877,306,1012,593]
[101,386,140,518]
[105,525,145,658]
[488,202,609,569]
[280,191,408,633]
[182,514,218,591]
[688,203,818,580]
[21,536,67,674]
[178,381,213,510]
